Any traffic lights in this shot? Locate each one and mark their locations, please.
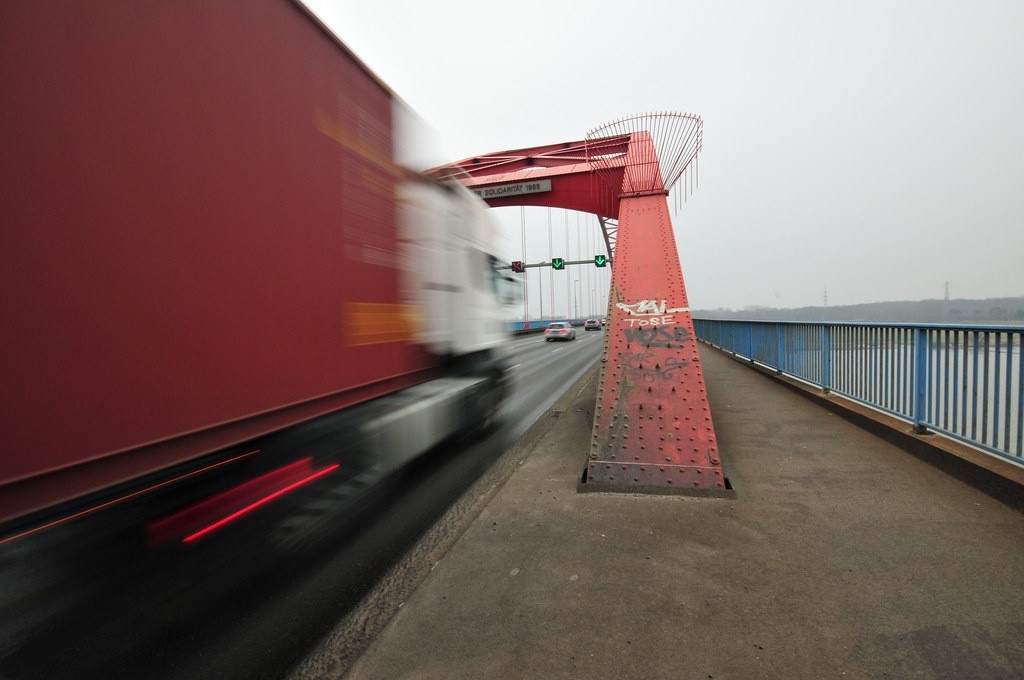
[552,258,563,269]
[595,255,607,268]
[512,261,523,272]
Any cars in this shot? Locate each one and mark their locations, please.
[602,317,607,325]
[585,319,602,330]
[545,322,577,341]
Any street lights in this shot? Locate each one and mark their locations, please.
[573,279,579,320]
[539,261,544,320]
